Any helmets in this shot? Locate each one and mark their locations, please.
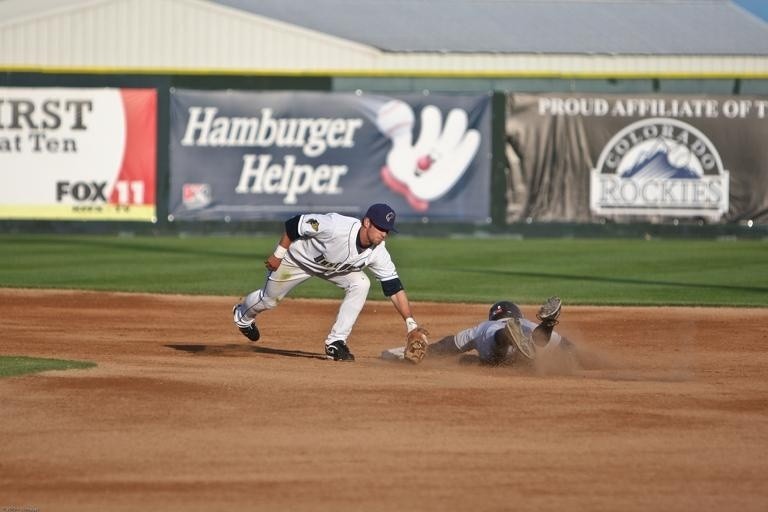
[489,301,522,321]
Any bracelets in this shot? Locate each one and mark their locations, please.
[405,317,417,333]
[273,244,287,259]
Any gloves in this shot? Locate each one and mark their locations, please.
[459,353,479,367]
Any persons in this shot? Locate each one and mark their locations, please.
[232,203,430,361]
[426,296,575,369]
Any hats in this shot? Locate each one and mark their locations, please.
[366,203,399,234]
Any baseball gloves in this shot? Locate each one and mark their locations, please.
[404,327,429,364]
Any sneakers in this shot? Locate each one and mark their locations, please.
[232,304,260,341]
[325,340,355,362]
[536,295,562,325]
[504,319,537,360]
